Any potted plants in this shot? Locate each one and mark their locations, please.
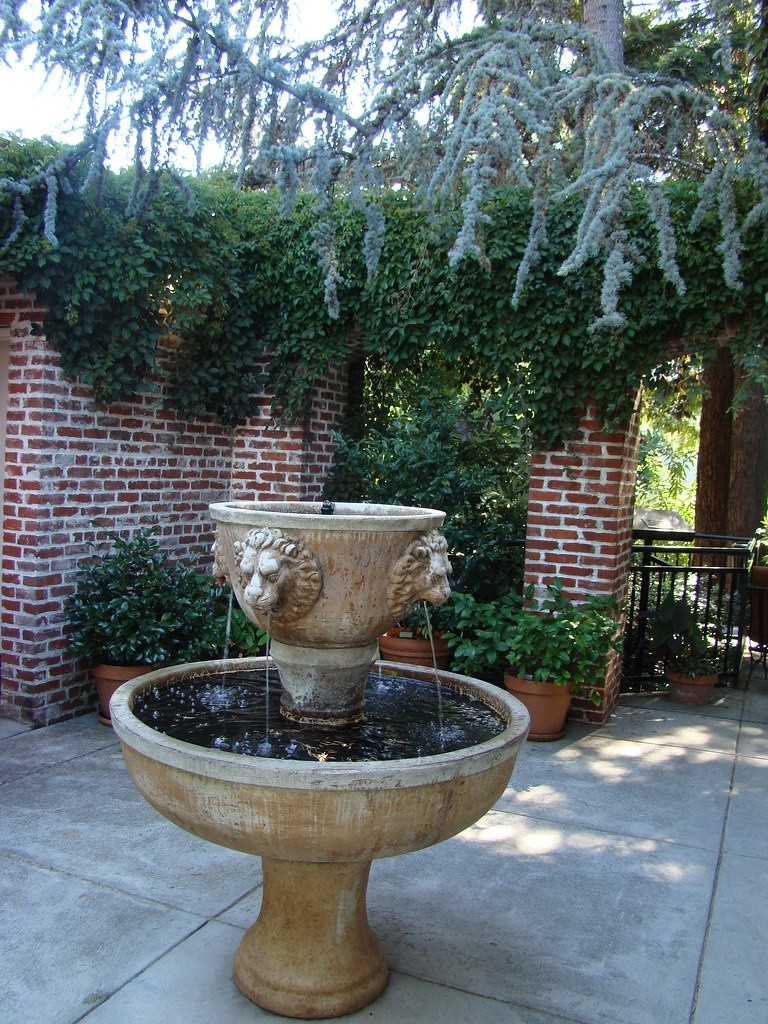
[746,515,768,646]
[438,577,629,742]
[378,604,456,670]
[634,588,724,707]
[63,513,224,728]
